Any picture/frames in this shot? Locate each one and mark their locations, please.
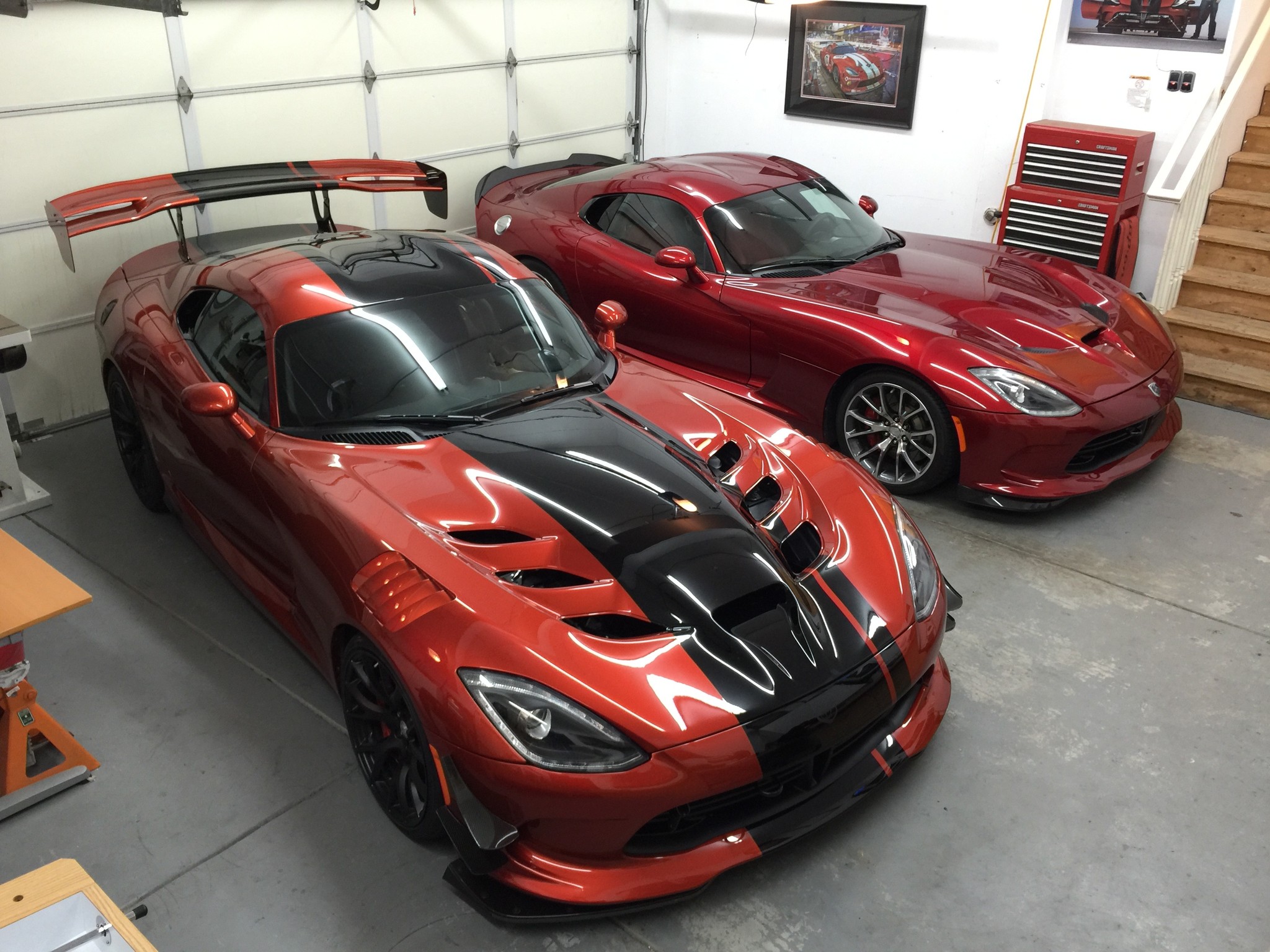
[784,0,926,129]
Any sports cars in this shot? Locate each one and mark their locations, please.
[474,151,1185,513]
[820,42,887,97]
[45,156,964,926]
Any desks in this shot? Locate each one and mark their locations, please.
[0,528,101,819]
